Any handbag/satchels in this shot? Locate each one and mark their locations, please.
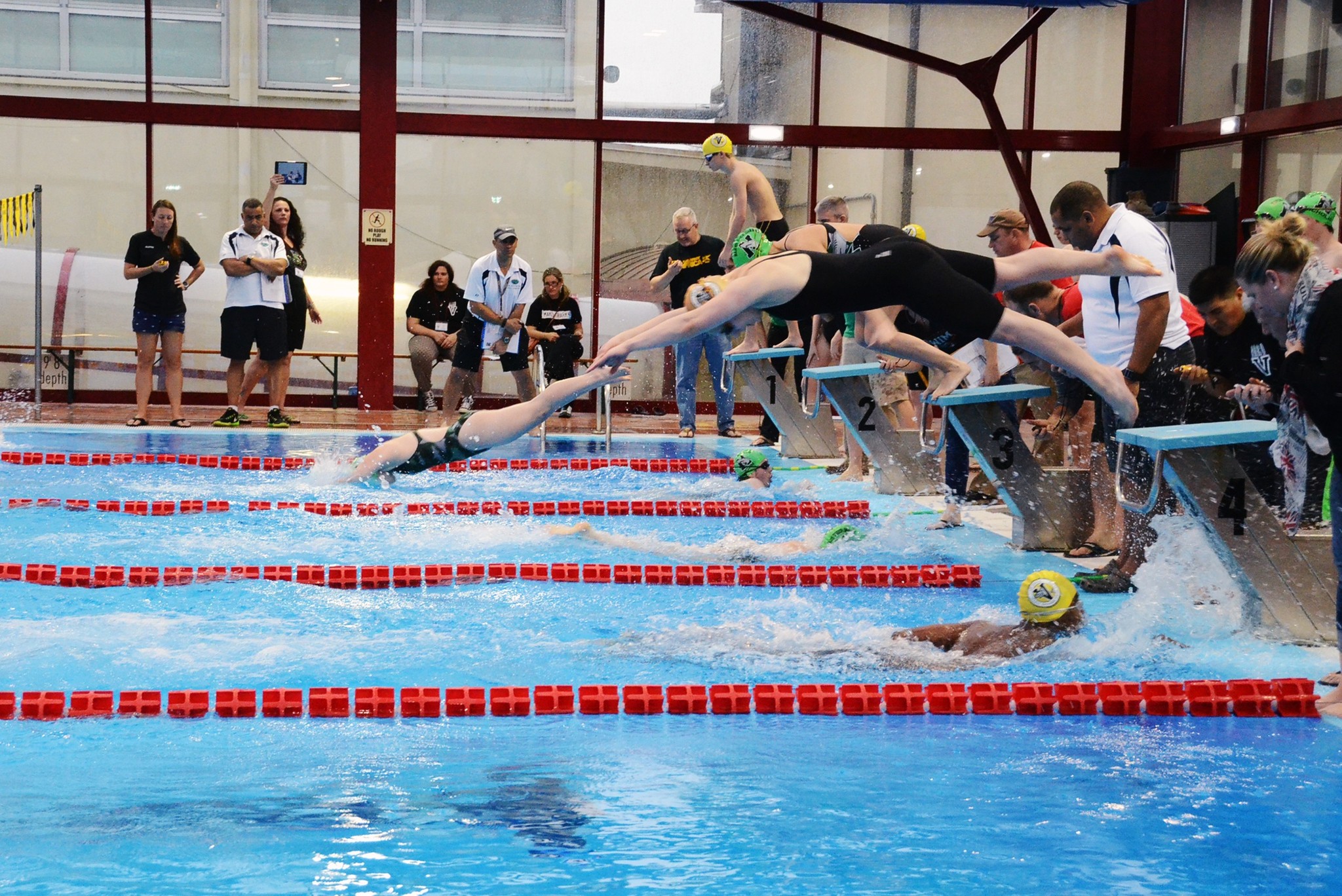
[527,339,539,356]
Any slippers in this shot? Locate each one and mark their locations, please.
[126,418,149,426]
[964,488,998,505]
[1062,541,1136,594]
[926,519,965,530]
[169,418,191,427]
[679,426,775,446]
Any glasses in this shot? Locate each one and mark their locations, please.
[544,279,560,287]
[1068,600,1083,612]
[1254,213,1274,220]
[1290,206,1336,215]
[705,152,718,162]
[756,461,770,471]
[676,224,695,233]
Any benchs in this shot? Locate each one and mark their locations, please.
[0,342,638,409]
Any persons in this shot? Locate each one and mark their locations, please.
[1227,213,1342,723]
[441,226,541,437]
[212,198,289,427]
[649,206,744,438]
[123,200,205,427]
[239,175,322,424]
[405,260,477,413]
[702,133,788,355]
[804,568,1084,674]
[525,267,583,418]
[731,449,773,494]
[283,171,302,183]
[588,235,1163,423]
[749,175,1341,593]
[730,223,964,399]
[342,366,632,487]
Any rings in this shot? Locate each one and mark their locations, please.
[276,181,279,183]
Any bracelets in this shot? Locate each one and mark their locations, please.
[501,318,507,328]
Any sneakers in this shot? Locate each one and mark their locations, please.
[458,395,474,414]
[422,390,438,412]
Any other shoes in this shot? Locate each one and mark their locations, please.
[211,405,300,427]
[558,405,572,418]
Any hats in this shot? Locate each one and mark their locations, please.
[733,228,773,269]
[977,208,1029,238]
[1255,197,1291,220]
[734,449,766,478]
[1019,570,1077,623]
[902,224,927,243]
[494,225,518,241]
[684,275,728,312]
[821,526,865,548]
[703,133,733,156]
[1294,192,1337,226]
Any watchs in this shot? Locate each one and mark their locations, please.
[246,255,255,264]
[501,336,510,344]
[182,281,188,290]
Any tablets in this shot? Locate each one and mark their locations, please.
[275,161,307,185]
[556,334,581,343]
